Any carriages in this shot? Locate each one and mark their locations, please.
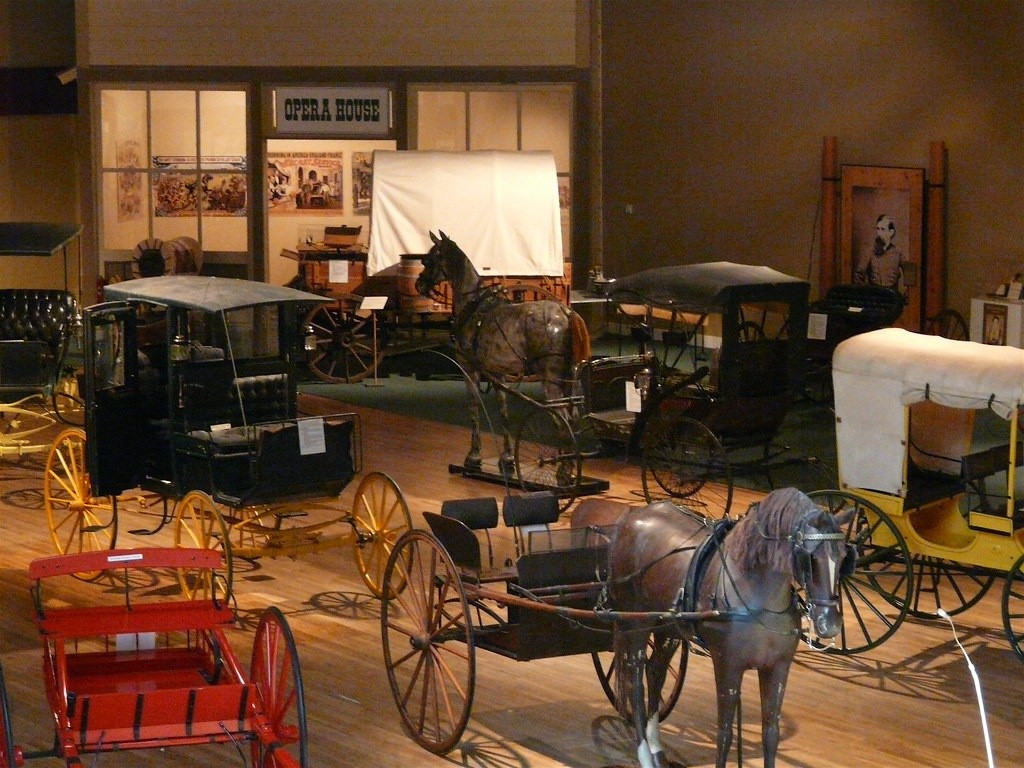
[380,488,851,768]
[414,224,810,497]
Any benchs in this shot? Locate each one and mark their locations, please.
[441,488,559,583]
[812,281,905,326]
[0,288,73,365]
[583,353,662,425]
[29,548,234,636]
[184,373,300,459]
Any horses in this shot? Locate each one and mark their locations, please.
[601,487,846,768]
[414,229,594,487]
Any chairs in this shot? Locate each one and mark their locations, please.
[422,511,520,586]
[903,440,967,512]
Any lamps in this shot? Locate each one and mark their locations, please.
[634,368,660,400]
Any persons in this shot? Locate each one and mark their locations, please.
[269,171,287,202]
[854,214,911,305]
